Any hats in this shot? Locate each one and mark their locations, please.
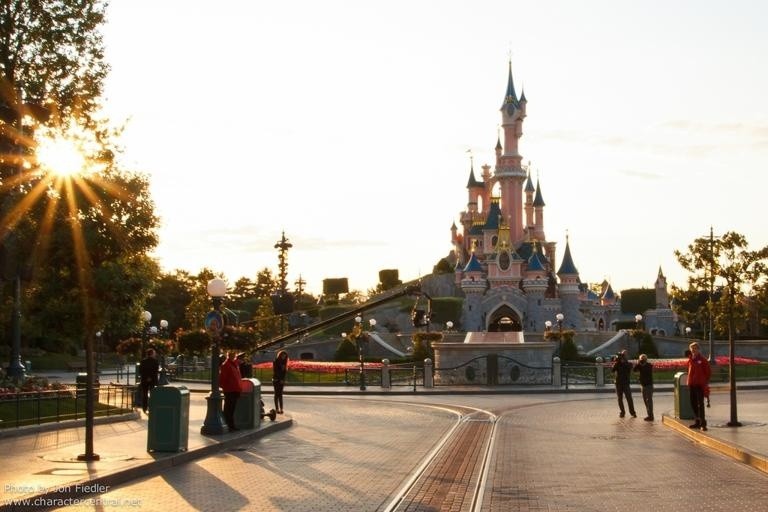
[237,352,249,360]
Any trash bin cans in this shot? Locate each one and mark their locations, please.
[147,384,190,453]
[232,378,261,429]
[673,371,700,419]
[76,372,100,403]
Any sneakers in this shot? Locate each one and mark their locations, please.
[620,412,653,421]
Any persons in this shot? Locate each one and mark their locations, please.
[633,354,654,421]
[686,342,711,431]
[611,350,637,418]
[139,349,158,413]
[272,350,289,414]
[218,350,249,433]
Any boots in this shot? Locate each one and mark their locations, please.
[689,419,708,431]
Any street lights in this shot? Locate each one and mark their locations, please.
[96,329,101,373]
[342,316,376,390]
[201,278,229,434]
[634,314,642,356]
[142,310,169,354]
[556,313,565,358]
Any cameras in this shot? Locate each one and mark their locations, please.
[615,352,622,359]
[640,360,646,364]
